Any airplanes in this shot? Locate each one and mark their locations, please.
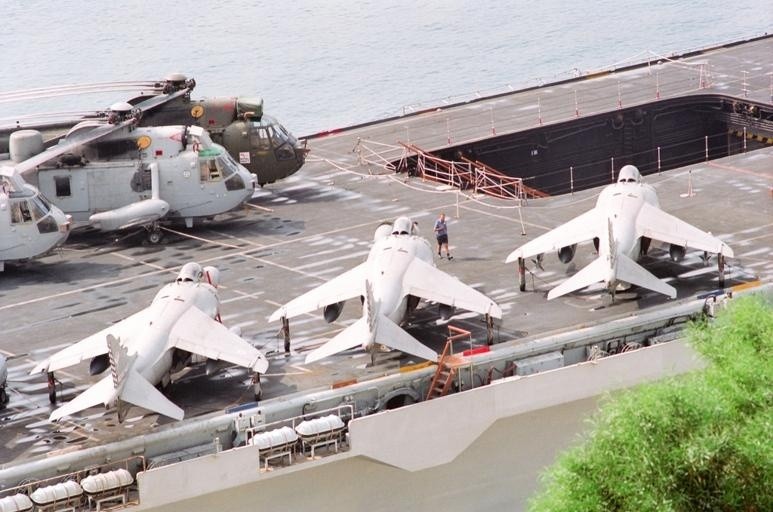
[503,163,736,301]
[26,261,269,424]
[266,216,503,366]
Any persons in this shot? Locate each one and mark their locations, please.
[433,213,453,261]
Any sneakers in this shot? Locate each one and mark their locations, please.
[448,256,453,260]
[439,254,442,259]
